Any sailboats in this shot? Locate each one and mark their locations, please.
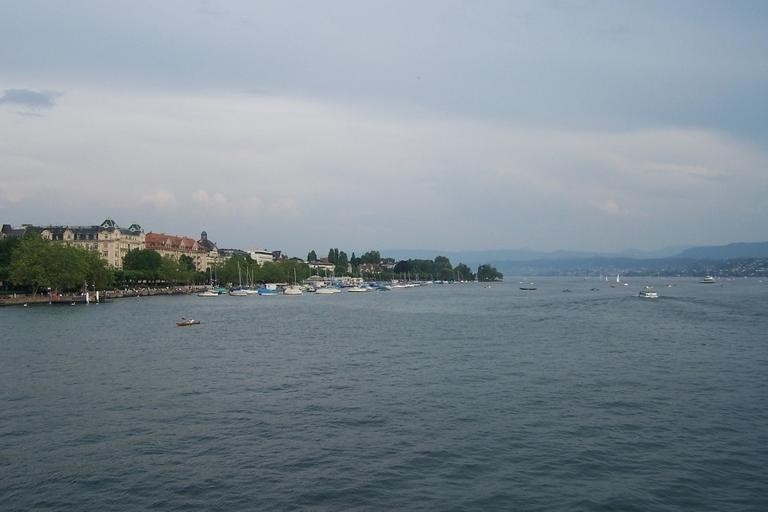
[616,273,620,283]
[193,265,472,296]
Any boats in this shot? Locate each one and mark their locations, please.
[637,285,658,299]
[519,281,537,286]
[699,275,717,284]
[520,288,537,291]
[175,318,201,327]
